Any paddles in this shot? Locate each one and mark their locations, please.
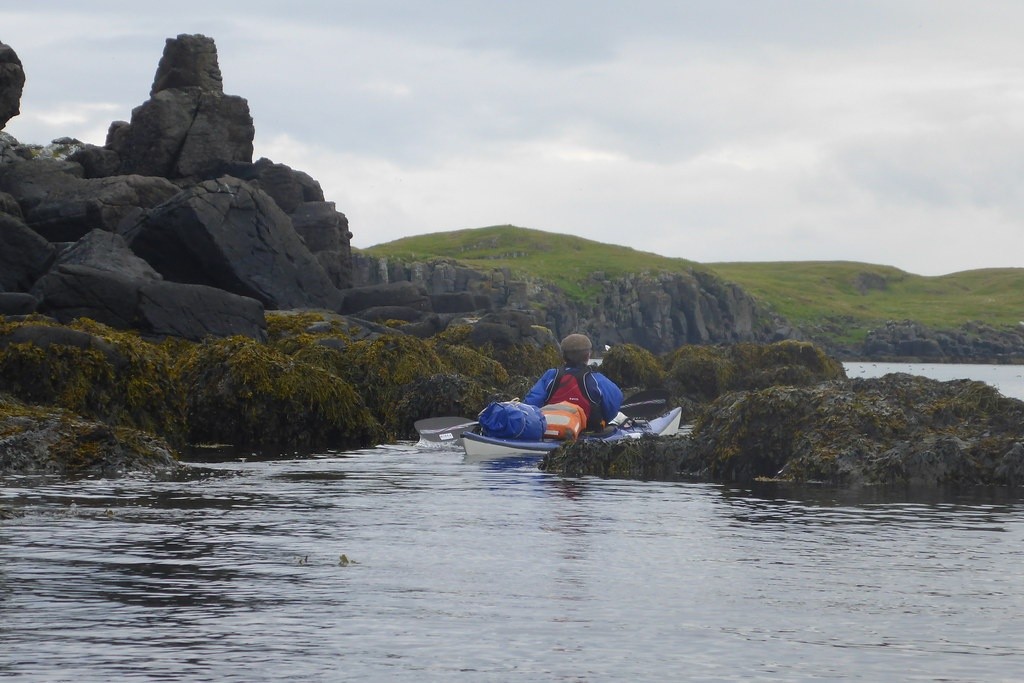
[413,388,668,443]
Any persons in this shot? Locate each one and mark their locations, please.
[523,334,623,430]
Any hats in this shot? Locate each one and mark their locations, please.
[560,333,592,351]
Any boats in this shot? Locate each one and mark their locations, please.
[459,407,682,456]
